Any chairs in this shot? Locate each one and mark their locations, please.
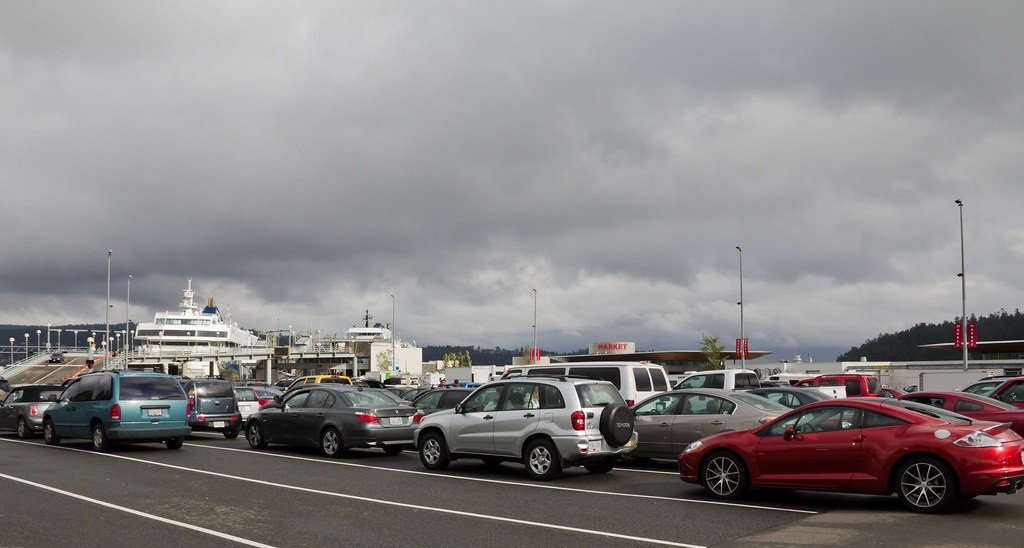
[849,412,868,428]
[49,395,57,401]
[509,392,526,410]
[682,400,694,414]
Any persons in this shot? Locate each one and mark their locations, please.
[484,387,513,411]
[454,379,459,387]
[438,380,446,388]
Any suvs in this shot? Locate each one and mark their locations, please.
[414,374,638,482]
[42,368,192,452]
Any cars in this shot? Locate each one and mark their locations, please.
[244,386,425,459]
[617,388,814,467]
[61,362,1024,436]
[0,376,43,405]
[677,397,1024,513]
[0,385,66,439]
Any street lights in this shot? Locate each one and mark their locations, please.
[109,337,114,361]
[24,334,30,361]
[391,294,395,372]
[87,337,95,360]
[736,246,745,370]
[37,330,41,355]
[116,333,120,357]
[954,199,968,371]
[105,250,113,370]
[533,289,536,364]
[126,275,133,370]
[122,330,127,354]
[101,342,106,367]
[130,330,134,361]
[9,337,15,365]
